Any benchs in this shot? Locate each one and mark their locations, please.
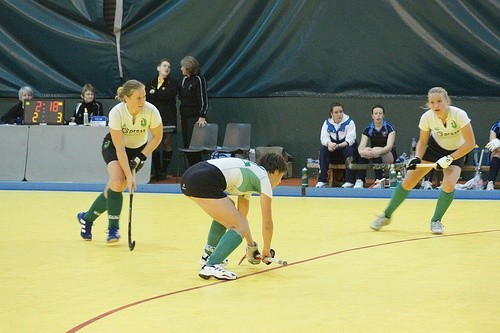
[307,159,490,189]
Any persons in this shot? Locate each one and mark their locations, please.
[0,86,34,125]
[368,87,476,234]
[64,84,103,125]
[486,121,500,190]
[178,56,209,170]
[315,102,359,188]
[180,153,288,280]
[353,105,397,189]
[77,80,163,244]
[145,59,181,182]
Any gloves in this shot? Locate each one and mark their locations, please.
[434,155,454,171]
[406,157,421,170]
[129,154,144,173]
[245,241,261,266]
[485,138,500,151]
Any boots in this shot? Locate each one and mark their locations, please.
[161,150,172,179]
[150,150,160,181]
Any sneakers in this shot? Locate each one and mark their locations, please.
[77,212,92,240]
[429,220,444,234]
[198,264,236,280]
[199,254,228,267]
[369,213,391,230]
[107,226,120,243]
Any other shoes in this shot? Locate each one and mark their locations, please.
[352,179,364,189]
[341,182,354,188]
[316,182,326,188]
[486,181,495,191]
[372,178,385,189]
[423,181,432,190]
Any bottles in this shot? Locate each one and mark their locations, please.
[411,138,417,154]
[302,166,308,188]
[84,108,88,125]
[383,163,402,189]
[473,167,483,190]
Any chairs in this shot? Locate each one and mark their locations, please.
[175,123,218,184]
[212,122,252,158]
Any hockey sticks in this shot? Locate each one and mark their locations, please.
[127,167,139,250]
[477,149,485,172]
[345,156,440,170]
[238,253,289,268]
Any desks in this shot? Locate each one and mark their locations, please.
[0,123,175,184]
